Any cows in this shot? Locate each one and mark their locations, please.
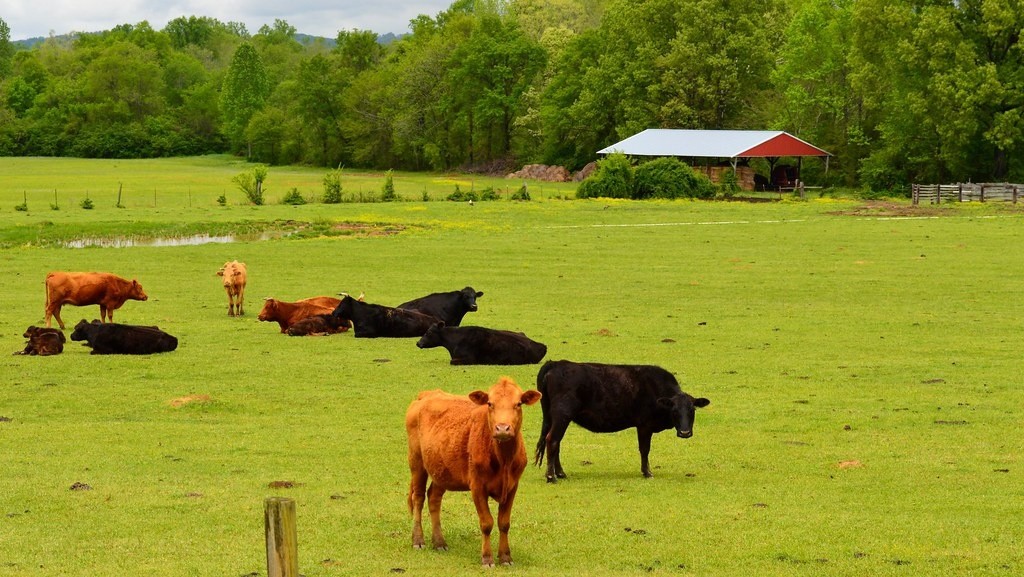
[14,272,178,357]
[215,259,710,567]
